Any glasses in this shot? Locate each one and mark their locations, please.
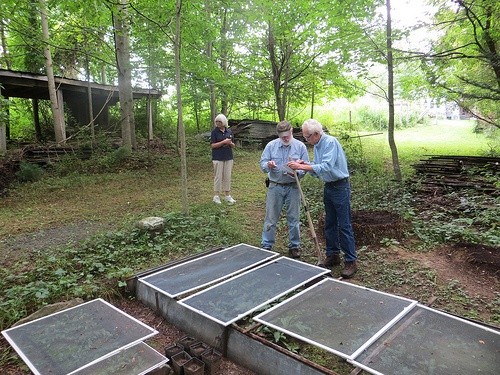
[302,133,312,138]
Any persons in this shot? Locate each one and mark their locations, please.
[210,113,237,206]
[285,118,359,278]
[259,119,310,260]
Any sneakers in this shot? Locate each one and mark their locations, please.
[213,196,221,205]
[341,261,357,278]
[291,248,300,258]
[225,195,236,204]
[316,257,341,267]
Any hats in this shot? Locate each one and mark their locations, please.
[277,121,291,138]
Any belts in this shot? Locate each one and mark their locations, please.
[325,178,349,184]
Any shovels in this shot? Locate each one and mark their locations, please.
[289,157,333,277]
